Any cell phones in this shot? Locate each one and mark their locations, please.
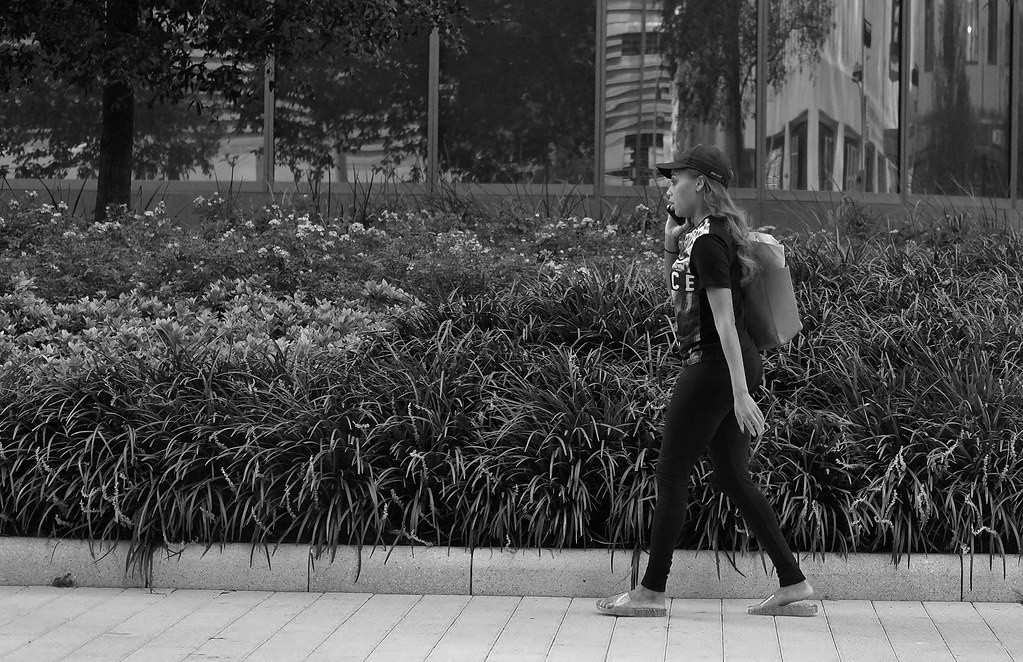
[667,204,685,225]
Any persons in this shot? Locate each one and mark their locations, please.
[596,144,819,617]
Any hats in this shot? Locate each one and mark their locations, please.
[655,144,733,189]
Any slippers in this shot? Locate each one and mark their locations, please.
[597,591,666,617]
[748,594,818,617]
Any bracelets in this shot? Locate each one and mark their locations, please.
[664,247,680,254]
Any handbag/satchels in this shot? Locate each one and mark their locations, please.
[740,232,803,351]
